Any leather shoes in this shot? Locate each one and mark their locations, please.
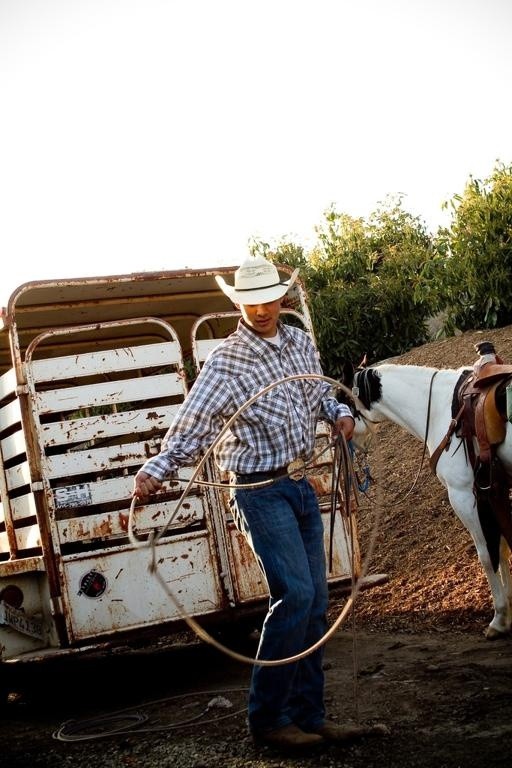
[251,723,324,747]
[307,720,366,739]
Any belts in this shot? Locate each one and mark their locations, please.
[273,459,306,481]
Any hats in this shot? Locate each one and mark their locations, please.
[215,254,300,305]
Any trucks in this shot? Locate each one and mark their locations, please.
[1,257,392,665]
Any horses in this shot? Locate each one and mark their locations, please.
[335,353,512,640]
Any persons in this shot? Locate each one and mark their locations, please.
[131,254,369,749]
